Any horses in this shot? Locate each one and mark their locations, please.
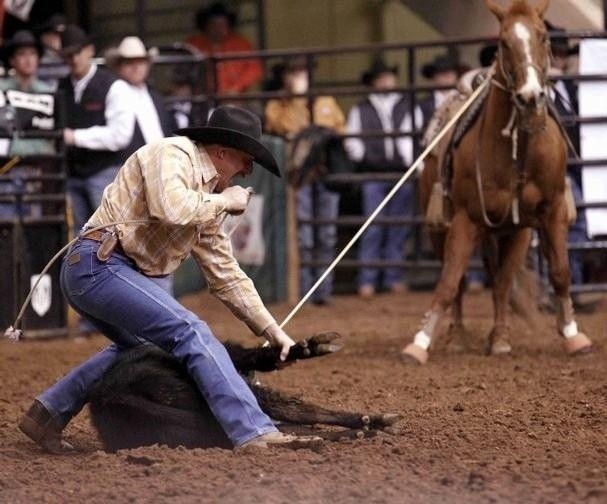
[400,1,595,365]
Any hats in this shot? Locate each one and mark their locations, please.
[3,32,42,55]
[40,12,74,33]
[264,53,316,90]
[422,56,462,75]
[59,26,95,55]
[172,106,281,178]
[363,62,396,81]
[107,35,159,60]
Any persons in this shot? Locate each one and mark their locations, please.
[264,50,345,307]
[37,16,69,77]
[345,57,424,299]
[420,51,488,294]
[182,1,263,106]
[53,27,135,340]
[17,105,325,450]
[479,21,601,314]
[162,71,208,127]
[105,34,174,162]
[0,30,67,341]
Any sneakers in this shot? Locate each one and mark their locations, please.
[235,433,324,450]
[18,401,73,454]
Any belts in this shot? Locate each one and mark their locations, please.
[82,227,106,244]
